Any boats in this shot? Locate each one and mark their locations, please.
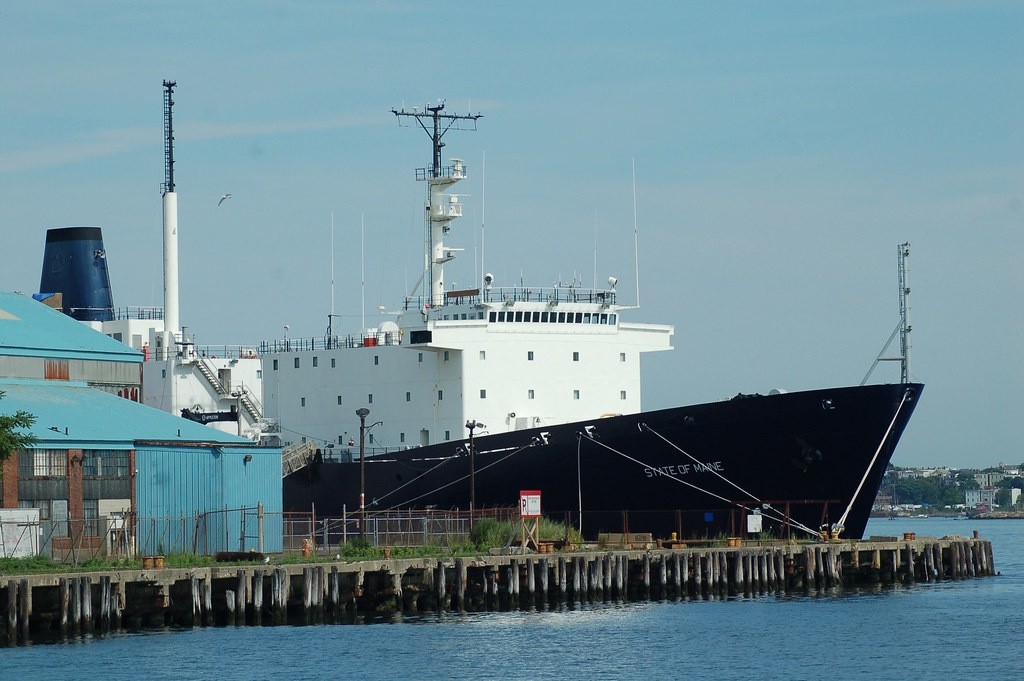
[40,80,925,541]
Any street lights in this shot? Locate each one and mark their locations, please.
[465,420,489,529]
[355,408,383,546]
